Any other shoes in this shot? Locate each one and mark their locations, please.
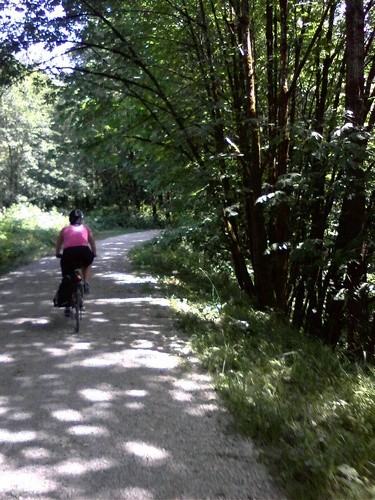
[65,309,71,317]
[84,282,92,294]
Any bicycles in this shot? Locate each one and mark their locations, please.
[55,251,97,333]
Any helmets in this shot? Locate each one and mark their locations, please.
[69,209,84,219]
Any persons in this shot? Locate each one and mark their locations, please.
[56,210,96,315]
